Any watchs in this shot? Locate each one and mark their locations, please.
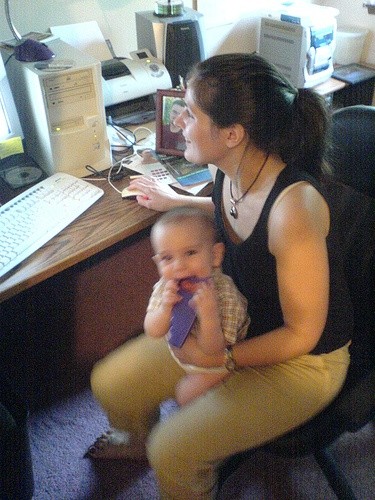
[223,344,242,376]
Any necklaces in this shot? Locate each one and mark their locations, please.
[228,154,272,220]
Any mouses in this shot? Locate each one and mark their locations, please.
[121,187,150,200]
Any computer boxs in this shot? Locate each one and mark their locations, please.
[4,37,113,178]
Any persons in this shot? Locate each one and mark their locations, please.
[79,50,358,500]
[162,101,189,150]
[141,205,261,407]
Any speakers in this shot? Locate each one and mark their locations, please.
[136,6,205,89]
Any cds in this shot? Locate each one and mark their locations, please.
[6,167,42,184]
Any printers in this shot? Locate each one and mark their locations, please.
[101,39,173,126]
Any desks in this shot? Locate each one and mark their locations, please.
[0,118,215,304]
[313,63,375,105]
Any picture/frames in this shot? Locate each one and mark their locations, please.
[156,89,186,156]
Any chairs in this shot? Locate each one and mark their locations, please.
[216,106,375,500]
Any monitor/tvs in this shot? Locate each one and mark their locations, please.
[0,53,24,144]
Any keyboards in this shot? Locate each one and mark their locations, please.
[0,172,105,278]
